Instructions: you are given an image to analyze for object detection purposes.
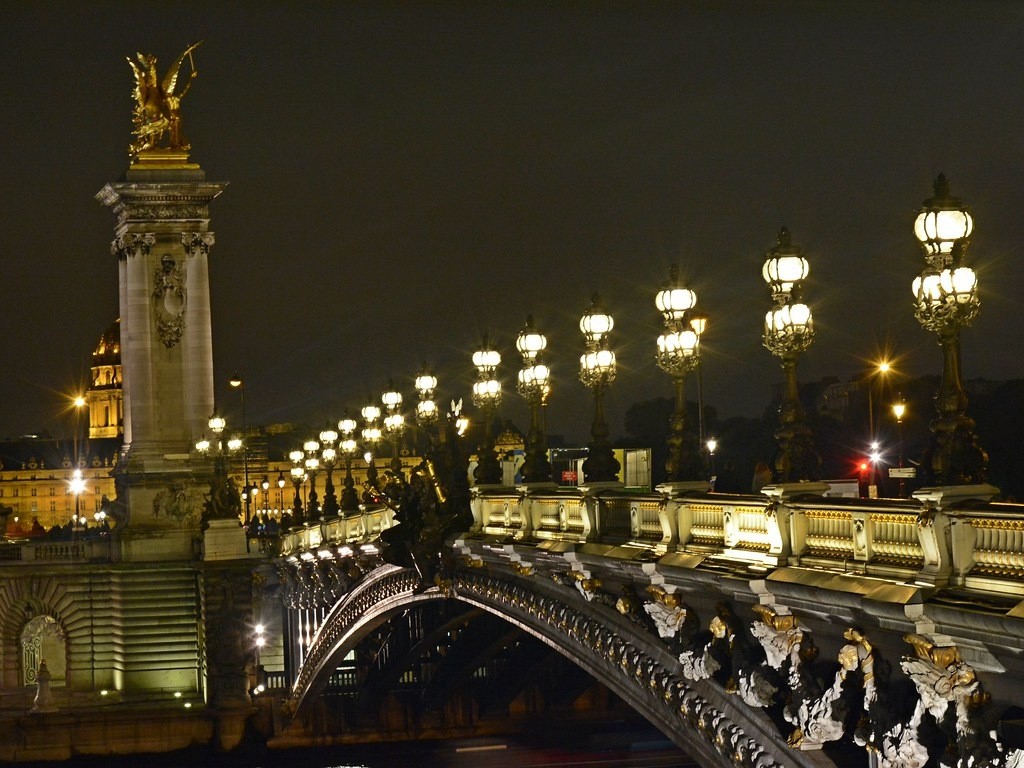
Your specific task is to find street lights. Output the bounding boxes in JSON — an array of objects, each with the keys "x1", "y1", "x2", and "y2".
[
  {"x1": 289, "y1": 449, "x2": 305, "y2": 525},
  {"x1": 228, "y1": 371, "x2": 251, "y2": 522},
  {"x1": 381, "y1": 382, "x2": 406, "y2": 487},
  {"x1": 361, "y1": 400, "x2": 383, "y2": 503},
  {"x1": 415, "y1": 363, "x2": 438, "y2": 456},
  {"x1": 277, "y1": 476, "x2": 285, "y2": 516},
  {"x1": 303, "y1": 438, "x2": 321, "y2": 521},
  {"x1": 905, "y1": 171, "x2": 999, "y2": 485},
  {"x1": 514, "y1": 313, "x2": 555, "y2": 482},
  {"x1": 577, "y1": 291, "x2": 624, "y2": 482},
  {"x1": 470, "y1": 331, "x2": 504, "y2": 483},
  {"x1": 338, "y1": 415, "x2": 360, "y2": 509},
  {"x1": 251, "y1": 484, "x2": 259, "y2": 520},
  {"x1": 654, "y1": 263, "x2": 703, "y2": 480},
  {"x1": 318, "y1": 425, "x2": 341, "y2": 520},
  {"x1": 195, "y1": 413, "x2": 242, "y2": 512},
  {"x1": 262, "y1": 477, "x2": 270, "y2": 517},
  {"x1": 759, "y1": 225, "x2": 823, "y2": 482},
  {"x1": 241, "y1": 489, "x2": 249, "y2": 525}
]
